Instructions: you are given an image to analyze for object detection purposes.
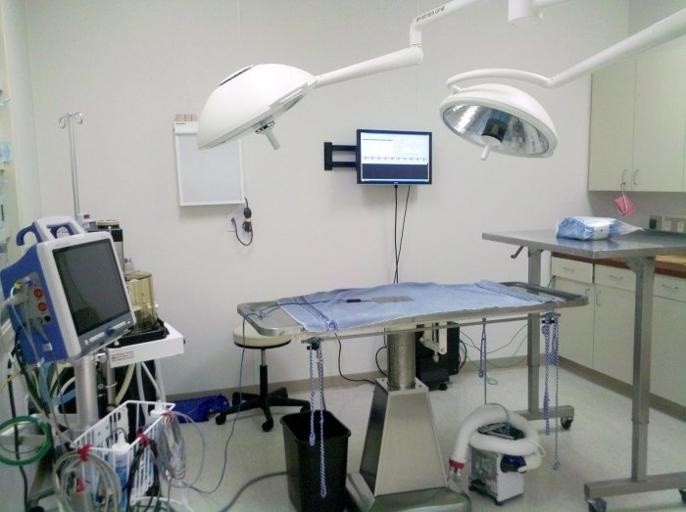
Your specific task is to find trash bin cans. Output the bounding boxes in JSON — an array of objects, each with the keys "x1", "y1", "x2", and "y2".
[{"x1": 280, "y1": 410, "x2": 351, "y2": 512}]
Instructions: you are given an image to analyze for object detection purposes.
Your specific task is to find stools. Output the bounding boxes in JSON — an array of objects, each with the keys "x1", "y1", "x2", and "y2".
[{"x1": 215, "y1": 326, "x2": 310, "y2": 432}]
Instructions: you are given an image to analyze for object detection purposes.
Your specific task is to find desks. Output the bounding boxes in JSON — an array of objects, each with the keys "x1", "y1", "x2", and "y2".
[{"x1": 482, "y1": 230, "x2": 685, "y2": 512}]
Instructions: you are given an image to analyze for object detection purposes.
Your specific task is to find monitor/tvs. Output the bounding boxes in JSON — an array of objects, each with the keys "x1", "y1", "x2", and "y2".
[
  {"x1": 0, "y1": 231, "x2": 136, "y2": 365},
  {"x1": 356, "y1": 128, "x2": 433, "y2": 185}
]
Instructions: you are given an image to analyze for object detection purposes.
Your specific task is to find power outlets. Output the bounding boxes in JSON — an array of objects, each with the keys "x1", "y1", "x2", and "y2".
[{"x1": 228, "y1": 213, "x2": 241, "y2": 232}]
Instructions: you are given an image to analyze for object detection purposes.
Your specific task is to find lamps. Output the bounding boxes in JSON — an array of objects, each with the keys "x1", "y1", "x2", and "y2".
[
  {"x1": 197, "y1": 0, "x2": 498, "y2": 152},
  {"x1": 440, "y1": 1, "x2": 686, "y2": 161}
]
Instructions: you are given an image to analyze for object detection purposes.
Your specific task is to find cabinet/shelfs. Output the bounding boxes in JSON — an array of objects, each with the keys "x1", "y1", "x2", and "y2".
[
  {"x1": 551, "y1": 255, "x2": 686, "y2": 407},
  {"x1": 587, "y1": 34, "x2": 686, "y2": 193}
]
